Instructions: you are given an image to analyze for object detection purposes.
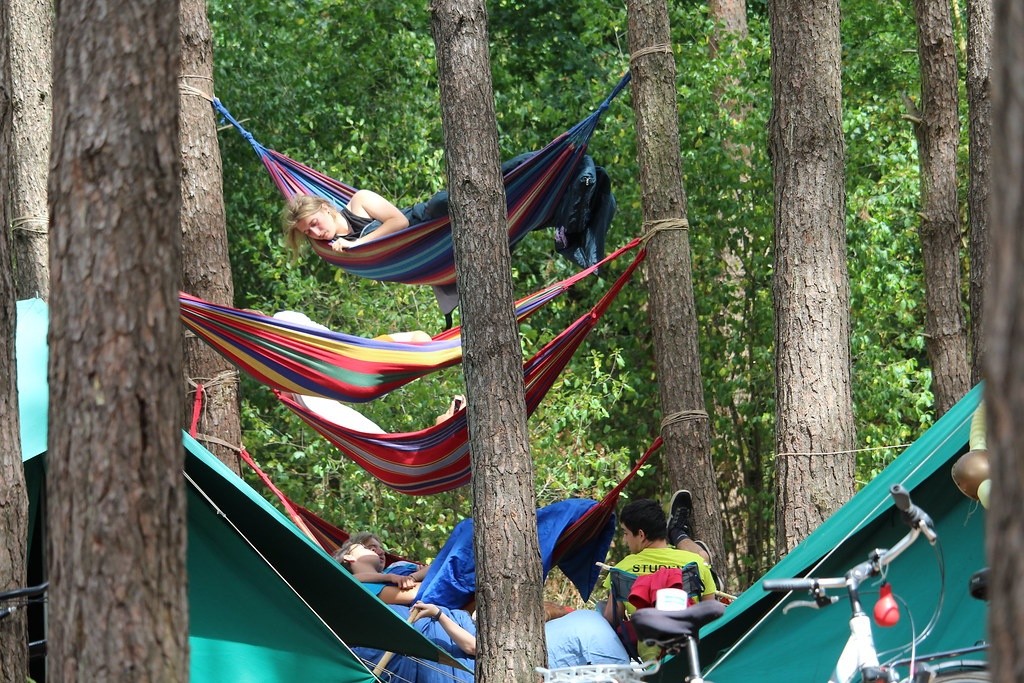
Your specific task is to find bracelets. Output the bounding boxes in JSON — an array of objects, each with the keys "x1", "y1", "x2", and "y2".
[
  {"x1": 431, "y1": 608, "x2": 443, "y2": 622},
  {"x1": 409, "y1": 574, "x2": 416, "y2": 582}
]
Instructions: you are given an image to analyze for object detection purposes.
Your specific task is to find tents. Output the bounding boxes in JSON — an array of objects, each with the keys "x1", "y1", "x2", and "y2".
[{"x1": 14, "y1": 294, "x2": 990, "y2": 683}]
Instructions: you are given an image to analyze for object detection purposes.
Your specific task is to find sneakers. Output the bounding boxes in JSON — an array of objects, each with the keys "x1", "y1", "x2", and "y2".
[
  {"x1": 666, "y1": 490, "x2": 692, "y2": 544},
  {"x1": 693, "y1": 541, "x2": 724, "y2": 592}
]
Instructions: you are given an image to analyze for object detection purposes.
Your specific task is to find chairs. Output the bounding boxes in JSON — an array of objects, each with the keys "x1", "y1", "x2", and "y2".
[{"x1": 610, "y1": 561, "x2": 704, "y2": 660}]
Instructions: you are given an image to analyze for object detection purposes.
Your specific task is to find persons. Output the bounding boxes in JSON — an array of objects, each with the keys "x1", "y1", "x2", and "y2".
[
  {"x1": 331, "y1": 530, "x2": 645, "y2": 683},
  {"x1": 604, "y1": 490, "x2": 723, "y2": 678},
  {"x1": 292, "y1": 394, "x2": 468, "y2": 433},
  {"x1": 284, "y1": 149, "x2": 542, "y2": 264},
  {"x1": 236, "y1": 309, "x2": 433, "y2": 343}
]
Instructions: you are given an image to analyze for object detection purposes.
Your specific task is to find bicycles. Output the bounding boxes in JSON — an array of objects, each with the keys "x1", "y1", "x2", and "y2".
[{"x1": 534, "y1": 484, "x2": 992, "y2": 683}]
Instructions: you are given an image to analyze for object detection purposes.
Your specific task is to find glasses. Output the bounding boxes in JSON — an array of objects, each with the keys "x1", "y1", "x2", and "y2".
[{"x1": 348, "y1": 542, "x2": 367, "y2": 555}]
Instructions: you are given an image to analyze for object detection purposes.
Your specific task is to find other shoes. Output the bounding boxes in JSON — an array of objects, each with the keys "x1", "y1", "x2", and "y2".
[{"x1": 970, "y1": 567, "x2": 991, "y2": 601}]
